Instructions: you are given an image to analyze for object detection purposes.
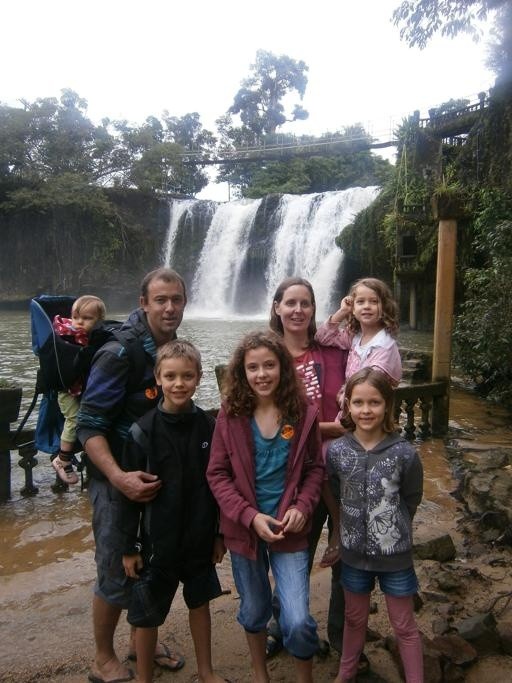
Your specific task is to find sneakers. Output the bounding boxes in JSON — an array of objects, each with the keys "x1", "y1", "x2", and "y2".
[{"x1": 52, "y1": 455, "x2": 78, "y2": 483}]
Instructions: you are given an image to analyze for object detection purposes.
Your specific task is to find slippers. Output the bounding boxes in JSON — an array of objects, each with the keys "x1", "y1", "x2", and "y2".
[
  {"x1": 266, "y1": 635, "x2": 283, "y2": 659},
  {"x1": 88, "y1": 668, "x2": 136, "y2": 683},
  {"x1": 357, "y1": 652, "x2": 369, "y2": 674},
  {"x1": 128, "y1": 643, "x2": 185, "y2": 671},
  {"x1": 316, "y1": 639, "x2": 330, "y2": 659}
]
